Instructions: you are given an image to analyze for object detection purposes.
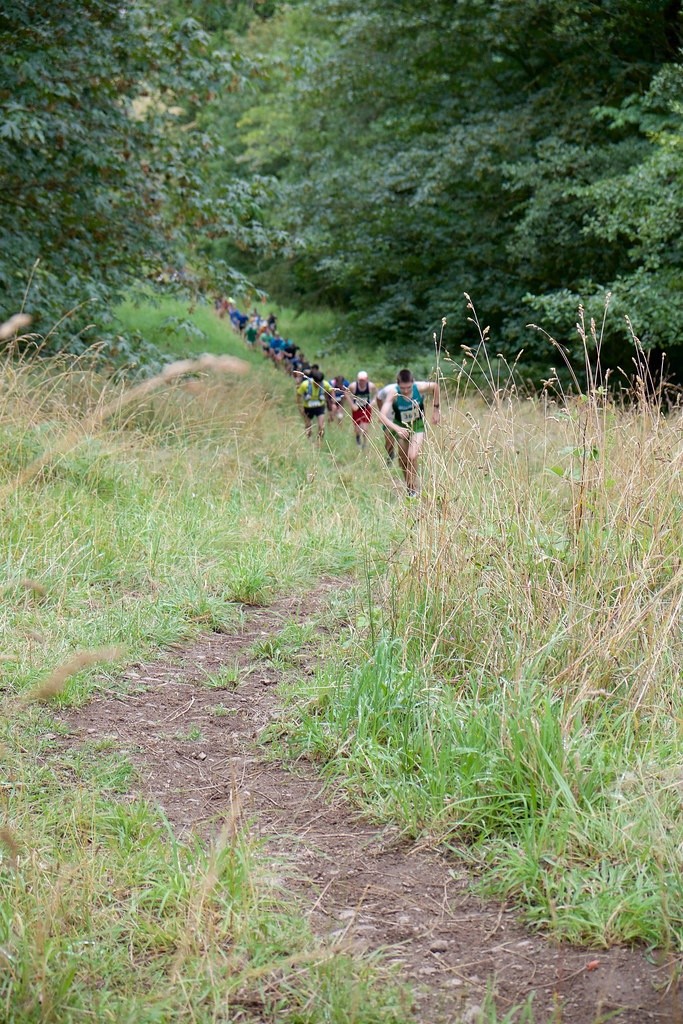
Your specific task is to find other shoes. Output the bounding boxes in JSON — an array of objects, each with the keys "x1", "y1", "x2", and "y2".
[
  {"x1": 356, "y1": 436, "x2": 361, "y2": 445},
  {"x1": 405, "y1": 490, "x2": 416, "y2": 499},
  {"x1": 385, "y1": 453, "x2": 395, "y2": 468}
]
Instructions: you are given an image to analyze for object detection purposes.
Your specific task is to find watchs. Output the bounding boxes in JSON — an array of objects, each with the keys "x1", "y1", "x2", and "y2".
[{"x1": 433, "y1": 404, "x2": 441, "y2": 409}]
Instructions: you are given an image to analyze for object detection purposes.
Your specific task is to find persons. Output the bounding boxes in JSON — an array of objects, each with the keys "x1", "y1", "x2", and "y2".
[
  {"x1": 374, "y1": 380, "x2": 408, "y2": 466},
  {"x1": 378, "y1": 368, "x2": 442, "y2": 498},
  {"x1": 214, "y1": 291, "x2": 378, "y2": 450}
]
